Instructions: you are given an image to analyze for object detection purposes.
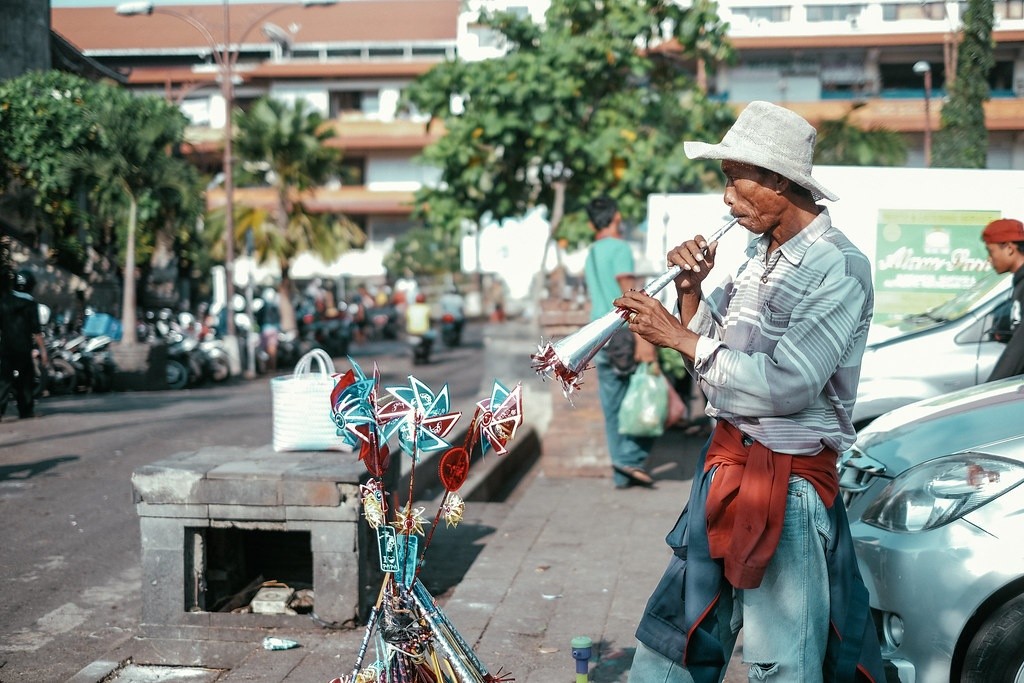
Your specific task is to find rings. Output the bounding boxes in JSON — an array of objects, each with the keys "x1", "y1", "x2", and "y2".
[{"x1": 629, "y1": 312, "x2": 638, "y2": 323}]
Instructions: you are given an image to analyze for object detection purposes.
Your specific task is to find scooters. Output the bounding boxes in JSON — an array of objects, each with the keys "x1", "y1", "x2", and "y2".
[
  {"x1": 439, "y1": 313, "x2": 466, "y2": 349},
  {"x1": 406, "y1": 316, "x2": 439, "y2": 367},
  {"x1": 1, "y1": 294, "x2": 397, "y2": 403}
]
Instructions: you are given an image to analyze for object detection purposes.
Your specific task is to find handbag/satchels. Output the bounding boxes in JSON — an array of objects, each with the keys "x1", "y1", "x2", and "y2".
[
  {"x1": 616, "y1": 361, "x2": 686, "y2": 437},
  {"x1": 608, "y1": 331, "x2": 636, "y2": 373}
]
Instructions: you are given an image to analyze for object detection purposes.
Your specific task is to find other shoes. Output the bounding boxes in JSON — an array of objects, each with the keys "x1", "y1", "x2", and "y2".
[{"x1": 614, "y1": 463, "x2": 653, "y2": 485}]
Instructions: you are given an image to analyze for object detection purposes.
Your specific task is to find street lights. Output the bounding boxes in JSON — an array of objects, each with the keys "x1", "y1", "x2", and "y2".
[{"x1": 115, "y1": 1, "x2": 339, "y2": 338}]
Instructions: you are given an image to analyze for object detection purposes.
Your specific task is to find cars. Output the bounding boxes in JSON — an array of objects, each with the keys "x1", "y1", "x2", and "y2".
[
  {"x1": 850, "y1": 268, "x2": 1024, "y2": 434},
  {"x1": 833, "y1": 375, "x2": 1024, "y2": 683}
]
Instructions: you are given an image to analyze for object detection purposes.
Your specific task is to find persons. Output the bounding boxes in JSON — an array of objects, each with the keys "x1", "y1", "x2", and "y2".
[
  {"x1": 611, "y1": 101, "x2": 873, "y2": 683},
  {"x1": 584, "y1": 196, "x2": 656, "y2": 489},
  {"x1": 981, "y1": 219, "x2": 1024, "y2": 343},
  {"x1": 264, "y1": 267, "x2": 466, "y2": 346},
  {"x1": 0, "y1": 270, "x2": 47, "y2": 417}
]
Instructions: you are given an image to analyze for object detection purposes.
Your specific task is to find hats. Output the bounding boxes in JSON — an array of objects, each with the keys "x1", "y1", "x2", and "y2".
[
  {"x1": 684, "y1": 99, "x2": 839, "y2": 203},
  {"x1": 982, "y1": 218, "x2": 1024, "y2": 243}
]
